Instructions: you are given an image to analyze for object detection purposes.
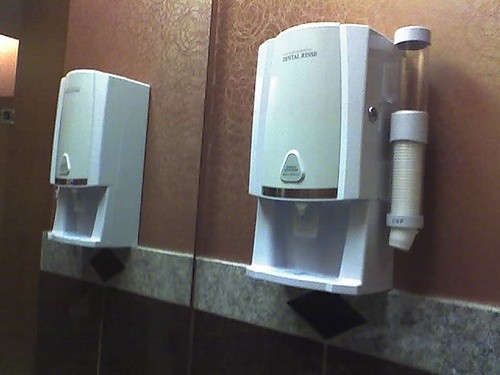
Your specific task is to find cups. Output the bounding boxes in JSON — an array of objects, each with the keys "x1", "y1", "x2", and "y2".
[{"x1": 388, "y1": 141, "x2": 424, "y2": 252}]
